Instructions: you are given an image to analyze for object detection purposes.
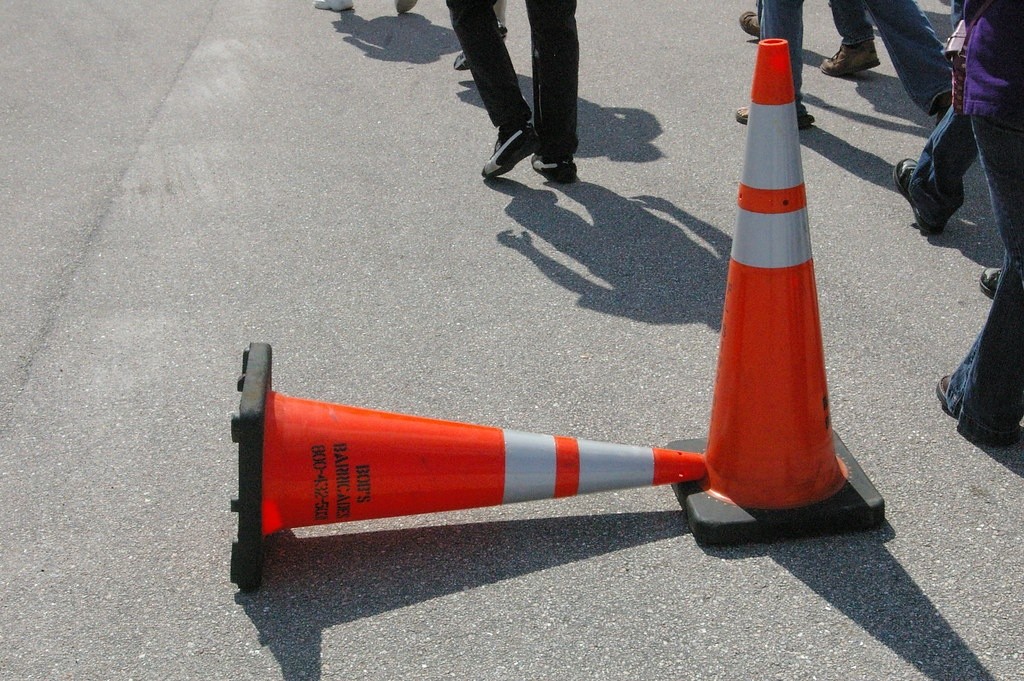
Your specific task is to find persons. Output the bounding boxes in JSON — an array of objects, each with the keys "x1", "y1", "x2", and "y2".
[
  {"x1": 446, "y1": 0, "x2": 579, "y2": 183},
  {"x1": 736, "y1": 0, "x2": 1024, "y2": 447},
  {"x1": 314, "y1": 0, "x2": 508, "y2": 74}
]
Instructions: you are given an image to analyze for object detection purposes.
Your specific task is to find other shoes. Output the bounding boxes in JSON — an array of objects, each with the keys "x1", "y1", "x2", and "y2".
[
  {"x1": 936, "y1": 374, "x2": 1022, "y2": 448},
  {"x1": 820, "y1": 38, "x2": 881, "y2": 76},
  {"x1": 935, "y1": 90, "x2": 952, "y2": 126},
  {"x1": 739, "y1": 11, "x2": 760, "y2": 37}
]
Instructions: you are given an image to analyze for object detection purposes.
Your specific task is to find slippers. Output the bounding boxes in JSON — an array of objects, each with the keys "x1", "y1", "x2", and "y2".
[{"x1": 735, "y1": 101, "x2": 815, "y2": 128}]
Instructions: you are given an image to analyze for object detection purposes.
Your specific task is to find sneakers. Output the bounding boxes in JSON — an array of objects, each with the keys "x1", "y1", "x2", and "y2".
[
  {"x1": 531, "y1": 154, "x2": 578, "y2": 184},
  {"x1": 893, "y1": 158, "x2": 947, "y2": 236},
  {"x1": 979, "y1": 266, "x2": 1002, "y2": 300},
  {"x1": 481, "y1": 123, "x2": 544, "y2": 179},
  {"x1": 313, "y1": 0, "x2": 354, "y2": 12},
  {"x1": 453, "y1": 16, "x2": 508, "y2": 71},
  {"x1": 394, "y1": 0, "x2": 419, "y2": 14}
]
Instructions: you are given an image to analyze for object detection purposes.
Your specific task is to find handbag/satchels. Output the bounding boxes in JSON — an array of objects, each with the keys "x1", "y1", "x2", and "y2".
[{"x1": 943, "y1": 19, "x2": 967, "y2": 116}]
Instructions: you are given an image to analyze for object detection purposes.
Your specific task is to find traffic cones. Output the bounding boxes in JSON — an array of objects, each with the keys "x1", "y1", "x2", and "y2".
[
  {"x1": 228, "y1": 343, "x2": 714, "y2": 587},
  {"x1": 655, "y1": 37, "x2": 886, "y2": 544}
]
